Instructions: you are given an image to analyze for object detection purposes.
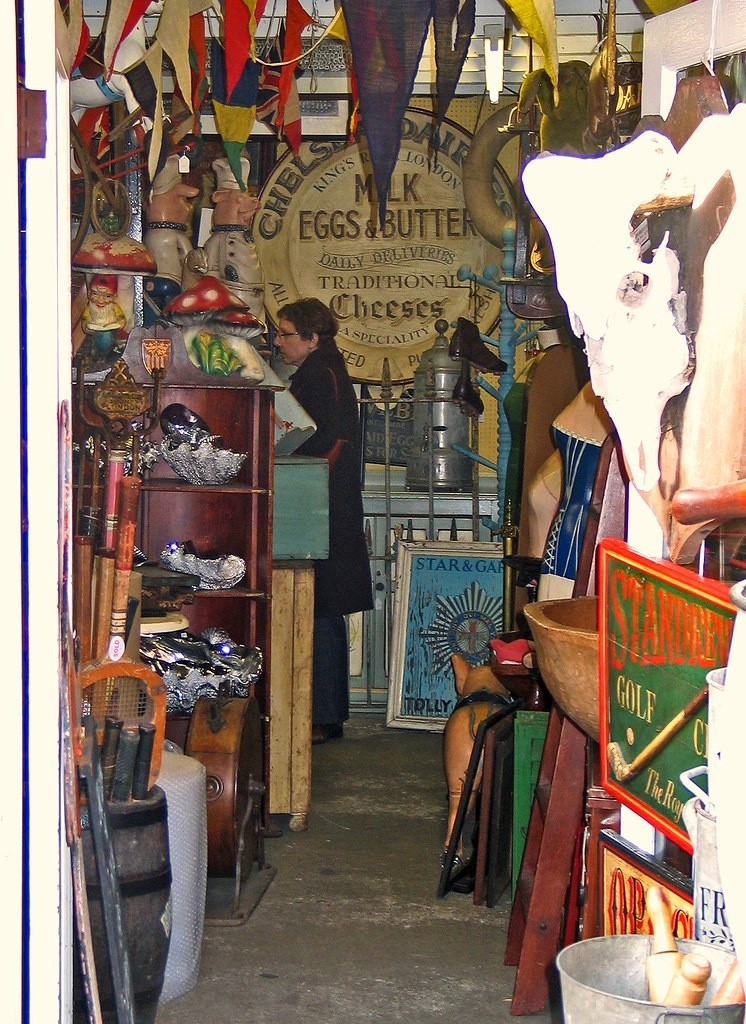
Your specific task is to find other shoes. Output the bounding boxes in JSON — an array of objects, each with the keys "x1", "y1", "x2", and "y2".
[{"x1": 312, "y1": 722, "x2": 344, "y2": 744}]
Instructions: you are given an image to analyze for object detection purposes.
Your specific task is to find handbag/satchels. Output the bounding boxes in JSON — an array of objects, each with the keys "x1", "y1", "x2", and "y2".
[{"x1": 294, "y1": 368, "x2": 350, "y2": 472}]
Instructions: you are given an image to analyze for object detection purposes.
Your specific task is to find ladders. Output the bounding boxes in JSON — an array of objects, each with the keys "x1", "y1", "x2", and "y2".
[{"x1": 502, "y1": 417, "x2": 637, "y2": 1016}]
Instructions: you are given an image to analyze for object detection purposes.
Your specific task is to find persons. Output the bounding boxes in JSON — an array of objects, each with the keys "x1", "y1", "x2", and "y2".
[
  {"x1": 81, "y1": 276, "x2": 127, "y2": 357},
  {"x1": 274, "y1": 297, "x2": 376, "y2": 743}
]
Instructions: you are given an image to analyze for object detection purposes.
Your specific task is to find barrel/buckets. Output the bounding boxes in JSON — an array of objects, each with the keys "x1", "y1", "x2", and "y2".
[{"x1": 557, "y1": 932, "x2": 746, "y2": 1024}]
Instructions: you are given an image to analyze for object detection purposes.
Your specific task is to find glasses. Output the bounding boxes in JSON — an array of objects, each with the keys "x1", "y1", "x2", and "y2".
[{"x1": 276, "y1": 329, "x2": 321, "y2": 340}]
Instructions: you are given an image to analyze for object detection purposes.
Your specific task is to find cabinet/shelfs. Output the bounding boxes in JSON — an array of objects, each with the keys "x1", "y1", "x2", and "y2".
[
  {"x1": 74, "y1": 324, "x2": 286, "y2": 929},
  {"x1": 344, "y1": 492, "x2": 500, "y2": 715}
]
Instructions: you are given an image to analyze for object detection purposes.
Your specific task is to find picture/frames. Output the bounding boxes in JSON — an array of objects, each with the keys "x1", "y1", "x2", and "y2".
[
  {"x1": 473, "y1": 713, "x2": 514, "y2": 908},
  {"x1": 386, "y1": 539, "x2": 505, "y2": 733}
]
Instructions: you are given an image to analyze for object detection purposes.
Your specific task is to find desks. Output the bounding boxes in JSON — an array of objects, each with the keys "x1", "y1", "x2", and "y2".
[{"x1": 490, "y1": 632, "x2": 553, "y2": 711}]
